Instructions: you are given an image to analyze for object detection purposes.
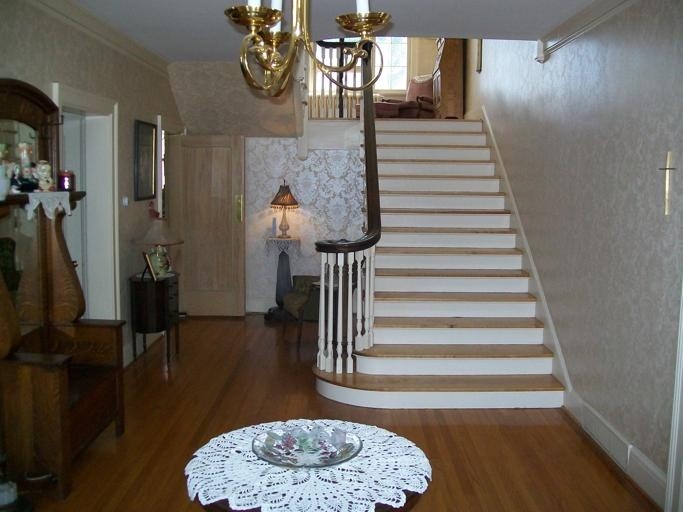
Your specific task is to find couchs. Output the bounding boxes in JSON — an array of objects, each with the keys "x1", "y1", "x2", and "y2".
[
  {"x1": 382, "y1": 74, "x2": 433, "y2": 119},
  {"x1": 356, "y1": 103, "x2": 400, "y2": 118}
]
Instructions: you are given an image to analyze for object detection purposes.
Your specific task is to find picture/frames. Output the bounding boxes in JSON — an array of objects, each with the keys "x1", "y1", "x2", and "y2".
[
  {"x1": 138, "y1": 251, "x2": 158, "y2": 283},
  {"x1": 133, "y1": 119, "x2": 157, "y2": 202}
]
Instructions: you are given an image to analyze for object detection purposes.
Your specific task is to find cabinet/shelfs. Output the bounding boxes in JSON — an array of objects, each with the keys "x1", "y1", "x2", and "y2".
[
  {"x1": 1, "y1": 191, "x2": 86, "y2": 218},
  {"x1": 432, "y1": 38, "x2": 464, "y2": 119},
  {"x1": 129, "y1": 273, "x2": 180, "y2": 363}
]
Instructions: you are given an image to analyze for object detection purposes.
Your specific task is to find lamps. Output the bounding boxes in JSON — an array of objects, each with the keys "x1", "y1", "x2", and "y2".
[
  {"x1": 269, "y1": 180, "x2": 300, "y2": 239},
  {"x1": 223, "y1": 0, "x2": 389, "y2": 105},
  {"x1": 130, "y1": 219, "x2": 186, "y2": 275}
]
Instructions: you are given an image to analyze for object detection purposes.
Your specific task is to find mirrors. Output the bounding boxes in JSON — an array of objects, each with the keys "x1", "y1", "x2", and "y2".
[{"x1": 0, "y1": 119, "x2": 46, "y2": 337}]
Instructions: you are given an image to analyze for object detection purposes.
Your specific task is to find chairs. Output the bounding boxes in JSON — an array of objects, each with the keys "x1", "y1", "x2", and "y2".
[{"x1": 282, "y1": 239, "x2": 366, "y2": 354}]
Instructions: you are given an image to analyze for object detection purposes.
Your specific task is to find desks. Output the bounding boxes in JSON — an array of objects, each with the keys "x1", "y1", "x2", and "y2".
[{"x1": 6, "y1": 319, "x2": 126, "y2": 501}]
